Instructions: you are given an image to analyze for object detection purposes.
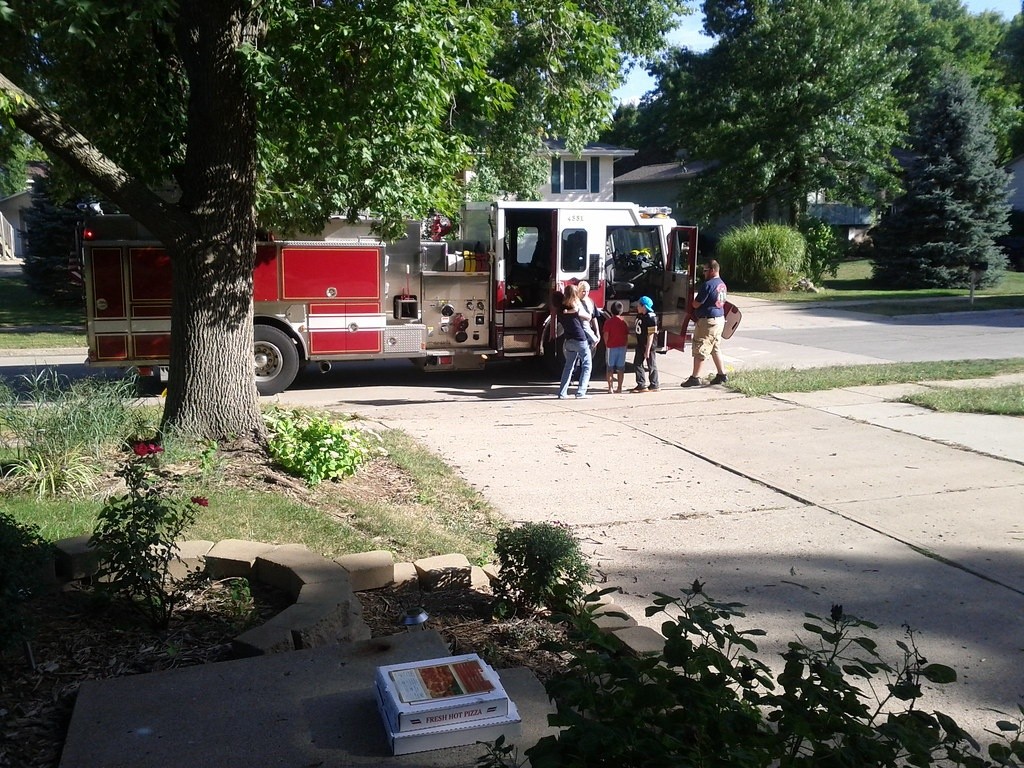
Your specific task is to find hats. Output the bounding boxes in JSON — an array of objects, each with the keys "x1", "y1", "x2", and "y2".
[{"x1": 640, "y1": 296, "x2": 653, "y2": 312}]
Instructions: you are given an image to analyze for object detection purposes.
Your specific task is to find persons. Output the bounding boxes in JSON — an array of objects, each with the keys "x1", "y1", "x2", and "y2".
[
  {"x1": 602, "y1": 301, "x2": 630, "y2": 394},
  {"x1": 680, "y1": 259, "x2": 729, "y2": 387},
  {"x1": 551, "y1": 280, "x2": 601, "y2": 399},
  {"x1": 630, "y1": 296, "x2": 661, "y2": 393}
]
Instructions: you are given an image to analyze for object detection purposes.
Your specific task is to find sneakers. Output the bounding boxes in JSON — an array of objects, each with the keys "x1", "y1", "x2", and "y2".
[
  {"x1": 681, "y1": 376, "x2": 700, "y2": 387},
  {"x1": 710, "y1": 373, "x2": 727, "y2": 385}
]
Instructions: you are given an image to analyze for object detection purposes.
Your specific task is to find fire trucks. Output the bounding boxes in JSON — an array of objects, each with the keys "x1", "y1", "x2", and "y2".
[{"x1": 78, "y1": 200, "x2": 699, "y2": 396}]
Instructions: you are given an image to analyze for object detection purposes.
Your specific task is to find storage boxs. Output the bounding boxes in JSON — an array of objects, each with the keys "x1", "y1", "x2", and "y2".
[
  {"x1": 373, "y1": 681, "x2": 522, "y2": 755},
  {"x1": 375, "y1": 651, "x2": 508, "y2": 732}
]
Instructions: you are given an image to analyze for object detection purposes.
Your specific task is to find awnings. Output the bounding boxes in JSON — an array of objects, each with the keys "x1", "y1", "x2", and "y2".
[{"x1": 807, "y1": 203, "x2": 873, "y2": 227}]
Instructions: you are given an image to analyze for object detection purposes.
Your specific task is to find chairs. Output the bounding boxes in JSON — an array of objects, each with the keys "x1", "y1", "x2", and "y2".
[{"x1": 606, "y1": 253, "x2": 634, "y2": 291}]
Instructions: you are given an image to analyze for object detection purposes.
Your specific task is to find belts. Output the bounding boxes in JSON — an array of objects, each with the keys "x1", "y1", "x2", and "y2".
[{"x1": 699, "y1": 316, "x2": 714, "y2": 318}]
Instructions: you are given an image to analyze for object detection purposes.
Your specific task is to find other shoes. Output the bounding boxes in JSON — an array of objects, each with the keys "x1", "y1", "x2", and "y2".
[
  {"x1": 559, "y1": 394, "x2": 575, "y2": 399},
  {"x1": 630, "y1": 388, "x2": 649, "y2": 392},
  {"x1": 575, "y1": 393, "x2": 592, "y2": 398},
  {"x1": 648, "y1": 388, "x2": 660, "y2": 391}
]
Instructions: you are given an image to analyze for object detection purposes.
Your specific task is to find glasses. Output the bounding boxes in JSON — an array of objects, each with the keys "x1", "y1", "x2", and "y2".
[{"x1": 704, "y1": 268, "x2": 716, "y2": 273}]
